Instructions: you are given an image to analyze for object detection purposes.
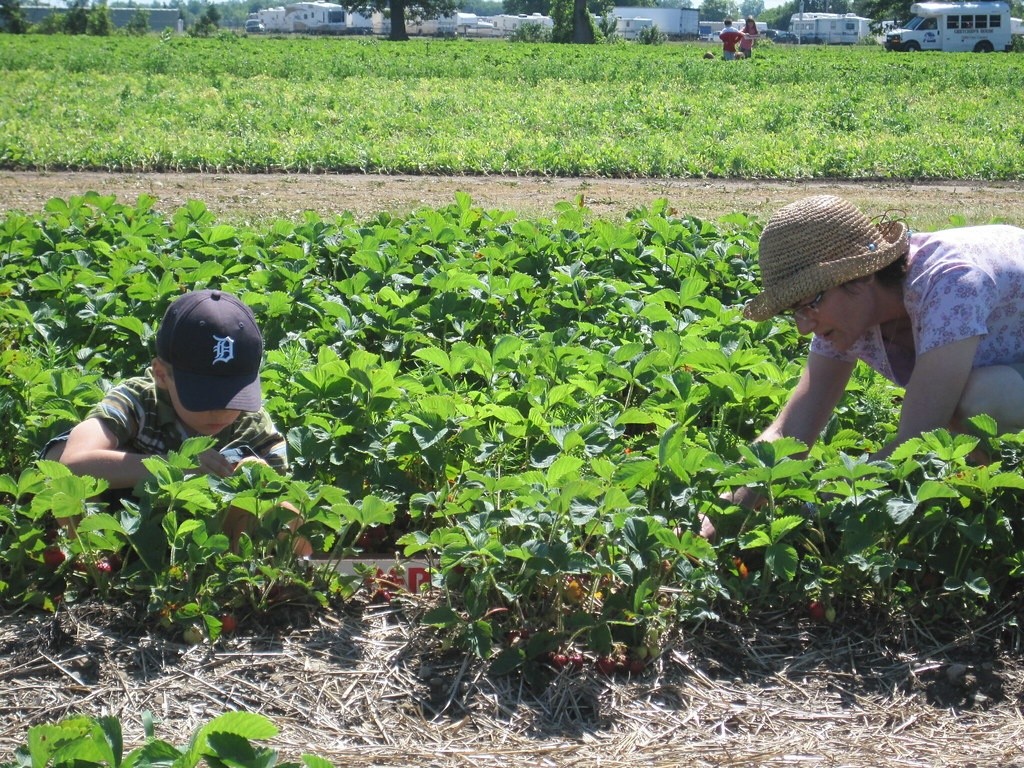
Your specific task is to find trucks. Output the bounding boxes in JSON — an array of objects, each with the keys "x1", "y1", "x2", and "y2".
[{"x1": 789, "y1": 16, "x2": 875, "y2": 45}]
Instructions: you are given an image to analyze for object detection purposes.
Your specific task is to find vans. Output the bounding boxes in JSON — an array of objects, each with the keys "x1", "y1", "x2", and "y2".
[{"x1": 884, "y1": 1, "x2": 1013, "y2": 53}]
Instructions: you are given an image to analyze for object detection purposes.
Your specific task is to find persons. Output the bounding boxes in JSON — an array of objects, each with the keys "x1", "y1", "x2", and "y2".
[
  {"x1": 719, "y1": 20, "x2": 743, "y2": 61},
  {"x1": 44, "y1": 288, "x2": 289, "y2": 499},
  {"x1": 738, "y1": 19, "x2": 758, "y2": 59},
  {"x1": 701, "y1": 194, "x2": 1023, "y2": 464}
]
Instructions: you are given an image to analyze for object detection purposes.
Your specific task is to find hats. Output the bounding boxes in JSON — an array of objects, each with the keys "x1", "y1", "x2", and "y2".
[
  {"x1": 157, "y1": 290, "x2": 262, "y2": 412},
  {"x1": 743, "y1": 195, "x2": 912, "y2": 322}
]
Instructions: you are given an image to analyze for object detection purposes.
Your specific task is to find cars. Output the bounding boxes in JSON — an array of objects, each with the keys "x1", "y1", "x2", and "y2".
[
  {"x1": 773, "y1": 32, "x2": 799, "y2": 45},
  {"x1": 707, "y1": 30, "x2": 724, "y2": 44},
  {"x1": 800, "y1": 32, "x2": 827, "y2": 45},
  {"x1": 760, "y1": 29, "x2": 779, "y2": 40},
  {"x1": 245, "y1": 18, "x2": 264, "y2": 33}
]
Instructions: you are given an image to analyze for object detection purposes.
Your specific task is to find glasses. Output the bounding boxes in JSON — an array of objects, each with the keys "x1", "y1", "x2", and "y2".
[{"x1": 777, "y1": 290, "x2": 824, "y2": 324}]
